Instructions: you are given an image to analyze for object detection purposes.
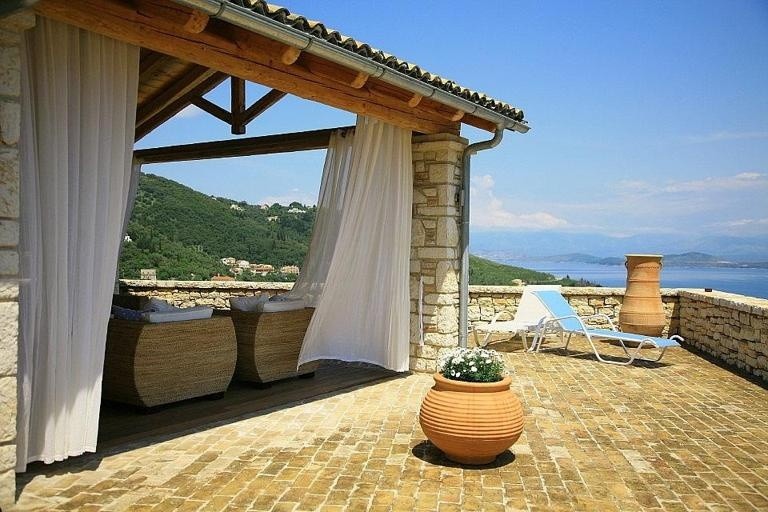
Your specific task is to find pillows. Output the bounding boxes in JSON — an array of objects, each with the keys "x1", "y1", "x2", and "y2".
[
  {"x1": 112, "y1": 296, "x2": 213, "y2": 322},
  {"x1": 229, "y1": 291, "x2": 306, "y2": 312}
]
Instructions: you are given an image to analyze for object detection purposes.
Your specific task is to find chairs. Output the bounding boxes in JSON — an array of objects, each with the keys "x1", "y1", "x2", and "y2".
[
  {"x1": 531, "y1": 290, "x2": 685, "y2": 365},
  {"x1": 215, "y1": 307, "x2": 319, "y2": 390},
  {"x1": 472, "y1": 284, "x2": 562, "y2": 351},
  {"x1": 103, "y1": 313, "x2": 238, "y2": 414}
]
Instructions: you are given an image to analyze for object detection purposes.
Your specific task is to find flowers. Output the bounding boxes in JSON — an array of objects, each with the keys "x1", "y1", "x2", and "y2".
[{"x1": 436, "y1": 346, "x2": 510, "y2": 382}]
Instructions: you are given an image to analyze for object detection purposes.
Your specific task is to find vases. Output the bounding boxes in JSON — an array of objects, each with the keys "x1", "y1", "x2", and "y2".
[
  {"x1": 419, "y1": 371, "x2": 525, "y2": 465},
  {"x1": 618, "y1": 253, "x2": 667, "y2": 347}
]
